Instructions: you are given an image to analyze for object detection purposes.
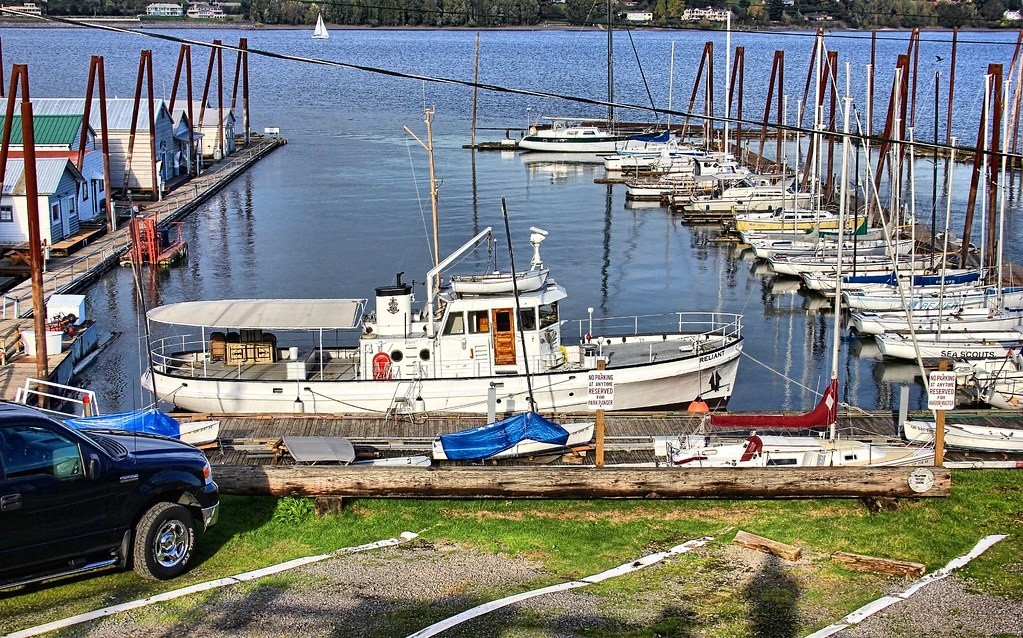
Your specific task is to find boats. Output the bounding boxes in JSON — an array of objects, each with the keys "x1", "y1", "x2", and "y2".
[
  {"x1": 906, "y1": 420, "x2": 1023, "y2": 455},
  {"x1": 122, "y1": 210, "x2": 189, "y2": 267}
]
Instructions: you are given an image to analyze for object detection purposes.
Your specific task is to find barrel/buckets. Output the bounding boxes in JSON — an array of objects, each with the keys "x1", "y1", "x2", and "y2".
[{"x1": 289, "y1": 346, "x2": 298, "y2": 360}]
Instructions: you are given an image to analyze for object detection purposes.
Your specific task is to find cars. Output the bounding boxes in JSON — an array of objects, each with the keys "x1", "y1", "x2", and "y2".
[{"x1": 0, "y1": 399, "x2": 220, "y2": 592}]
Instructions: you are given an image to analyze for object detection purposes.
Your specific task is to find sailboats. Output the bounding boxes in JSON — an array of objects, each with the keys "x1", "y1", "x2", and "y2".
[
  {"x1": 141, "y1": 82, "x2": 745, "y2": 408},
  {"x1": 517, "y1": 0, "x2": 1023, "y2": 410},
  {"x1": 311, "y1": 13, "x2": 330, "y2": 39},
  {"x1": 431, "y1": 196, "x2": 596, "y2": 464},
  {"x1": 656, "y1": 63, "x2": 946, "y2": 467}
]
